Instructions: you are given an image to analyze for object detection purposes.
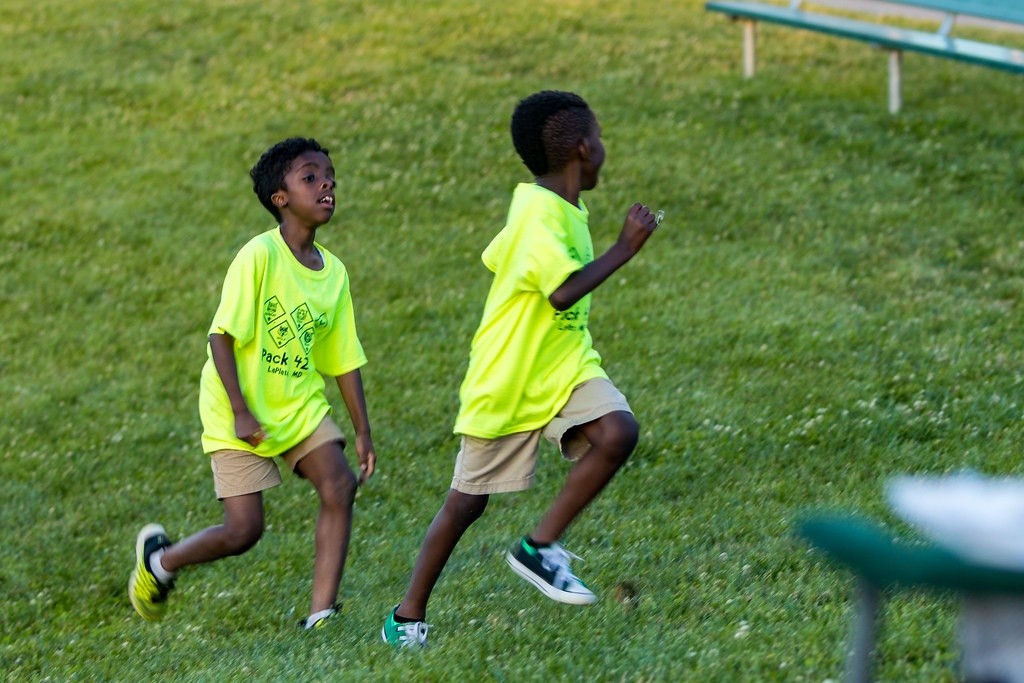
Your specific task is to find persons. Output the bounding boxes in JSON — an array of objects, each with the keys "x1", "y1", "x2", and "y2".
[
  {"x1": 381, "y1": 90, "x2": 658, "y2": 643},
  {"x1": 128, "y1": 135, "x2": 375, "y2": 631}
]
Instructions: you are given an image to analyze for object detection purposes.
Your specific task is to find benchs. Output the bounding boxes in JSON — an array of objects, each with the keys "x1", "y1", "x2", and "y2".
[{"x1": 704, "y1": 0, "x2": 1024, "y2": 113}]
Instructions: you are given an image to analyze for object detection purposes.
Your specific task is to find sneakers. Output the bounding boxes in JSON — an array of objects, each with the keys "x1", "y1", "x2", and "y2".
[
  {"x1": 128, "y1": 523, "x2": 178, "y2": 621},
  {"x1": 504, "y1": 531, "x2": 598, "y2": 605},
  {"x1": 298, "y1": 602, "x2": 343, "y2": 629},
  {"x1": 381, "y1": 604, "x2": 434, "y2": 648}
]
